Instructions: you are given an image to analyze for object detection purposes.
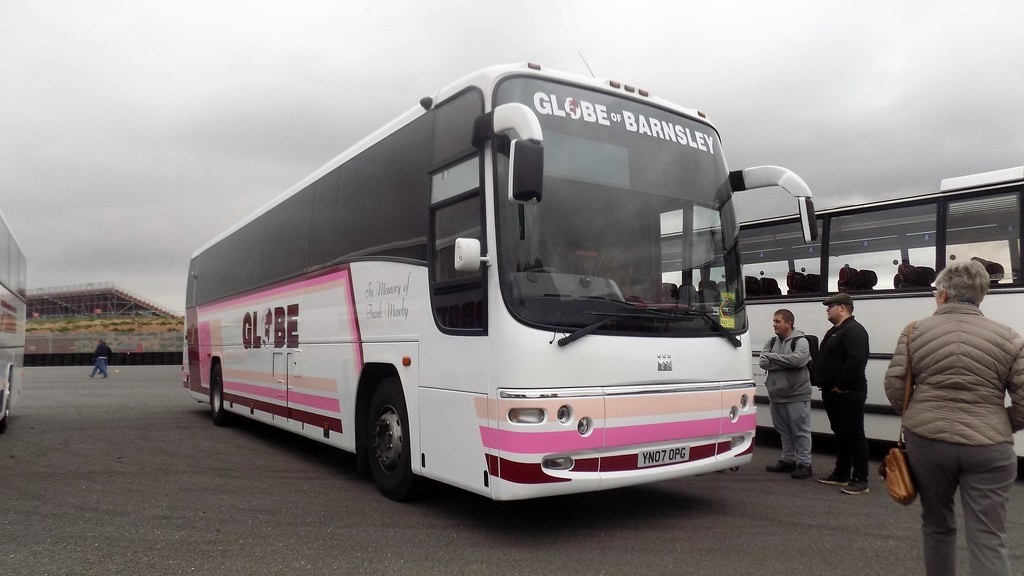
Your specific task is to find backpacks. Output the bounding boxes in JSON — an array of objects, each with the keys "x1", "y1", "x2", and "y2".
[{"x1": 769, "y1": 335, "x2": 818, "y2": 386}]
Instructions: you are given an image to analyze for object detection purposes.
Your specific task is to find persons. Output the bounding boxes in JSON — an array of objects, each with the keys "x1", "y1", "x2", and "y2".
[
  {"x1": 89, "y1": 339, "x2": 113, "y2": 379},
  {"x1": 810, "y1": 291, "x2": 869, "y2": 495},
  {"x1": 760, "y1": 309, "x2": 815, "y2": 479},
  {"x1": 884, "y1": 259, "x2": 1024, "y2": 576}
]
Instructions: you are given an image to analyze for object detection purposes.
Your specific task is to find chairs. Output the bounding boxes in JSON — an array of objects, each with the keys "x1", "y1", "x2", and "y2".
[{"x1": 661, "y1": 256, "x2": 1005, "y2": 305}]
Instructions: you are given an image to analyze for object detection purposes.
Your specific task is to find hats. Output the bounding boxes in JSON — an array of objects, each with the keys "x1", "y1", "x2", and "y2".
[{"x1": 822, "y1": 293, "x2": 853, "y2": 306}]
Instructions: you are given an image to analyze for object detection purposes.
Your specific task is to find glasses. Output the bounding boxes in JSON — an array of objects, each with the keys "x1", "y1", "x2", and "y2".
[
  {"x1": 932, "y1": 290, "x2": 941, "y2": 296},
  {"x1": 828, "y1": 304, "x2": 840, "y2": 308}
]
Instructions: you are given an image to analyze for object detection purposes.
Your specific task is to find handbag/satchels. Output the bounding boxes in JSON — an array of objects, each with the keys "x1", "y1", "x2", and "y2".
[{"x1": 877, "y1": 448, "x2": 917, "y2": 507}]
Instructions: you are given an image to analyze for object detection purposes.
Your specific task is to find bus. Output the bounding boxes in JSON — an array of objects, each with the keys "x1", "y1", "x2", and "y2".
[
  {"x1": 182, "y1": 61, "x2": 817, "y2": 503},
  {"x1": 659, "y1": 166, "x2": 1024, "y2": 458},
  {"x1": 0, "y1": 211, "x2": 27, "y2": 431}
]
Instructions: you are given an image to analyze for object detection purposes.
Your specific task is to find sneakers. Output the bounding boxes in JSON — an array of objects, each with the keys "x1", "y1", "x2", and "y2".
[
  {"x1": 839, "y1": 479, "x2": 869, "y2": 494},
  {"x1": 791, "y1": 464, "x2": 813, "y2": 479},
  {"x1": 766, "y1": 460, "x2": 797, "y2": 473},
  {"x1": 818, "y1": 471, "x2": 848, "y2": 486}
]
